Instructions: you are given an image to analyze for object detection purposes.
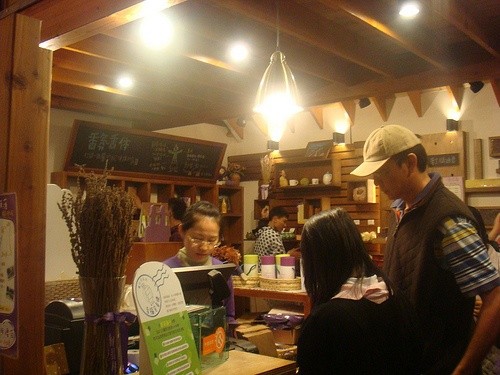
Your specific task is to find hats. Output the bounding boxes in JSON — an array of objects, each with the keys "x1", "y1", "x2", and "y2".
[{"x1": 350, "y1": 124, "x2": 421, "y2": 177}]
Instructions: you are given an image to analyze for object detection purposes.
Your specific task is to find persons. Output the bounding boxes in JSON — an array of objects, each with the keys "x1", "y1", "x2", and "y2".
[
  {"x1": 349, "y1": 124, "x2": 500, "y2": 375},
  {"x1": 255, "y1": 207, "x2": 301, "y2": 273},
  {"x1": 163, "y1": 197, "x2": 235, "y2": 320},
  {"x1": 467, "y1": 205, "x2": 500, "y2": 375},
  {"x1": 296, "y1": 207, "x2": 433, "y2": 375}
]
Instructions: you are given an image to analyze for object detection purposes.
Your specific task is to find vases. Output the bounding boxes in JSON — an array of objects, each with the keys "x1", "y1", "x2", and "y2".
[
  {"x1": 216, "y1": 180, "x2": 225, "y2": 185},
  {"x1": 230, "y1": 173, "x2": 241, "y2": 187},
  {"x1": 76, "y1": 276, "x2": 126, "y2": 375}
]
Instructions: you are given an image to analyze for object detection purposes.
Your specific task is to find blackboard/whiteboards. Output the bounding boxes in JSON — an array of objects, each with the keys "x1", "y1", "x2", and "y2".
[{"x1": 63, "y1": 118, "x2": 228, "y2": 183}]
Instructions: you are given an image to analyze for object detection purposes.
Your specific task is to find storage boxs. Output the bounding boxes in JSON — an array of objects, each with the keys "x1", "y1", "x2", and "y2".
[
  {"x1": 346, "y1": 179, "x2": 376, "y2": 204},
  {"x1": 304, "y1": 196, "x2": 330, "y2": 219},
  {"x1": 243, "y1": 329, "x2": 278, "y2": 358},
  {"x1": 274, "y1": 159, "x2": 342, "y2": 191},
  {"x1": 236, "y1": 324, "x2": 270, "y2": 339},
  {"x1": 188, "y1": 303, "x2": 230, "y2": 370},
  {"x1": 272, "y1": 328, "x2": 300, "y2": 345}
]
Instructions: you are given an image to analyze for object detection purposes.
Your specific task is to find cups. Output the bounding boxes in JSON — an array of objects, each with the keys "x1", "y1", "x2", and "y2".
[
  {"x1": 323, "y1": 171, "x2": 333, "y2": 184},
  {"x1": 290, "y1": 179, "x2": 299, "y2": 185},
  {"x1": 312, "y1": 179, "x2": 320, "y2": 184}
]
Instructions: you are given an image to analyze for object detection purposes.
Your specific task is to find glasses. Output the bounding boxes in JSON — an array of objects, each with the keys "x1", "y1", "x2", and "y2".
[{"x1": 185, "y1": 231, "x2": 218, "y2": 247}]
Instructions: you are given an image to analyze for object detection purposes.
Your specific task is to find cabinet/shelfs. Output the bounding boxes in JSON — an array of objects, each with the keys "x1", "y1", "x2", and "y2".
[{"x1": 50, "y1": 170, "x2": 245, "y2": 272}]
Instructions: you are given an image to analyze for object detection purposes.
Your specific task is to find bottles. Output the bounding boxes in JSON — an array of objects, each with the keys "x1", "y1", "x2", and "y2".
[
  {"x1": 221, "y1": 198, "x2": 227, "y2": 214},
  {"x1": 243, "y1": 254, "x2": 296, "y2": 279}
]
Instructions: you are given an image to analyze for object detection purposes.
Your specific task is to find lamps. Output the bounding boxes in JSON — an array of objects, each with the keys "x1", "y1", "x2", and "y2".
[
  {"x1": 252, "y1": 0, "x2": 304, "y2": 116},
  {"x1": 266, "y1": 140, "x2": 280, "y2": 151},
  {"x1": 332, "y1": 133, "x2": 345, "y2": 144},
  {"x1": 446, "y1": 119, "x2": 459, "y2": 132}
]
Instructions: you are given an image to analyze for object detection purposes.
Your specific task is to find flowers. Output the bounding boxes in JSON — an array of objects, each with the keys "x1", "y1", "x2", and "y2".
[
  {"x1": 227, "y1": 162, "x2": 246, "y2": 177},
  {"x1": 216, "y1": 165, "x2": 229, "y2": 181}
]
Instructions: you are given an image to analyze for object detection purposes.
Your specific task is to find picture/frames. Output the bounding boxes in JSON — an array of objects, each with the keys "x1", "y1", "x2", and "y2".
[
  {"x1": 488, "y1": 136, "x2": 500, "y2": 157},
  {"x1": 304, "y1": 140, "x2": 334, "y2": 159}
]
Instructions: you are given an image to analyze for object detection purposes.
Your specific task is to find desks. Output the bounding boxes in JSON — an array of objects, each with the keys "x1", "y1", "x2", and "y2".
[
  {"x1": 233, "y1": 286, "x2": 312, "y2": 321},
  {"x1": 127, "y1": 347, "x2": 298, "y2": 375}
]
